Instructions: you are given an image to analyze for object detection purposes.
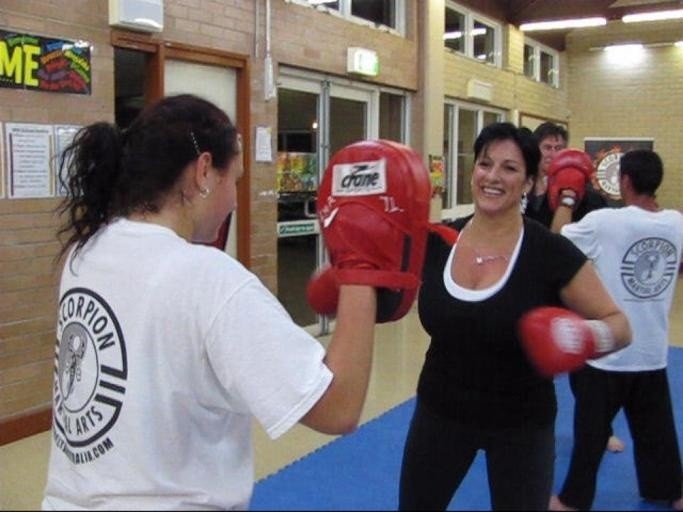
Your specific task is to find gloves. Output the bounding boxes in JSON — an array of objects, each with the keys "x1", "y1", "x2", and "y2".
[{"x1": 521, "y1": 306, "x2": 616, "y2": 378}]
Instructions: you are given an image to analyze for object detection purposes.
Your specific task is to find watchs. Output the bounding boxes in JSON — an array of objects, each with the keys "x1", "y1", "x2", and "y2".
[{"x1": 557, "y1": 194, "x2": 577, "y2": 211}]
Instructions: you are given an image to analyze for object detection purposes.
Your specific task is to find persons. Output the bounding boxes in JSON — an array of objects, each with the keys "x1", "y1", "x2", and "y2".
[
  {"x1": 548, "y1": 147, "x2": 682, "y2": 512},
  {"x1": 395, "y1": 120, "x2": 635, "y2": 511},
  {"x1": 520, "y1": 120, "x2": 624, "y2": 455},
  {"x1": 39, "y1": 93, "x2": 432, "y2": 511}
]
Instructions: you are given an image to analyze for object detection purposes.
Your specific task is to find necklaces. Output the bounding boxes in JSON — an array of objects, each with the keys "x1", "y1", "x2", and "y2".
[{"x1": 466, "y1": 214, "x2": 525, "y2": 266}]
